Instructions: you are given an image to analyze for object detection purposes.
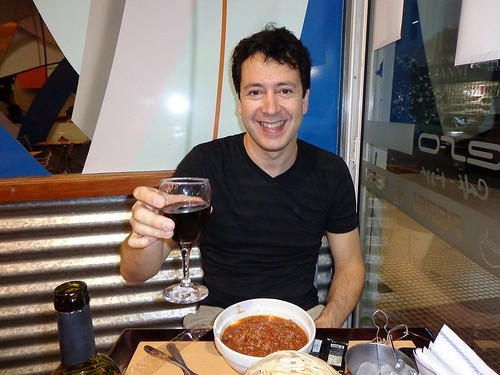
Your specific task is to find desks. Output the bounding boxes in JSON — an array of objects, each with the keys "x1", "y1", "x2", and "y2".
[{"x1": 106, "y1": 328, "x2": 435, "y2": 375}]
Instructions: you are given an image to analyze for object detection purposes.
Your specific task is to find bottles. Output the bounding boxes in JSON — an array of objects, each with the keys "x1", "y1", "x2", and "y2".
[{"x1": 51, "y1": 281, "x2": 122, "y2": 375}]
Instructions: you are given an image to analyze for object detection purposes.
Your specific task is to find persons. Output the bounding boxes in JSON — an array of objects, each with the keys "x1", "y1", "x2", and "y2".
[{"x1": 120, "y1": 28, "x2": 364, "y2": 330}]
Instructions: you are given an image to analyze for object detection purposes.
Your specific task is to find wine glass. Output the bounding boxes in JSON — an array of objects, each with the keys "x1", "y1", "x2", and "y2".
[{"x1": 156, "y1": 177, "x2": 212, "y2": 304}]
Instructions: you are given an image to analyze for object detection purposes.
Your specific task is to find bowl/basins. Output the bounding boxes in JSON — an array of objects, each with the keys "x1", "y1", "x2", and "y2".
[
  {"x1": 345, "y1": 343, "x2": 418, "y2": 375},
  {"x1": 213, "y1": 297, "x2": 316, "y2": 374}
]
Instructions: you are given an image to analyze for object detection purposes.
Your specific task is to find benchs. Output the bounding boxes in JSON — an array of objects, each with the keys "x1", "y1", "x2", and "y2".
[{"x1": 0, "y1": 169, "x2": 352, "y2": 375}]
